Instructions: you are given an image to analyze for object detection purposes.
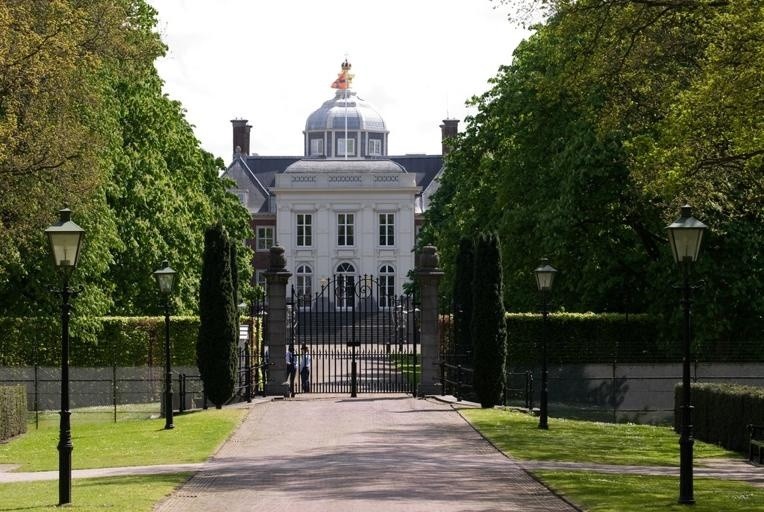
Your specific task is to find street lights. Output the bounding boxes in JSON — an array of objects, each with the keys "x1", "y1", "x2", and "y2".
[
  {"x1": 44, "y1": 208, "x2": 86, "y2": 504},
  {"x1": 532, "y1": 257, "x2": 558, "y2": 430},
  {"x1": 664, "y1": 204, "x2": 709, "y2": 506},
  {"x1": 153, "y1": 259, "x2": 177, "y2": 429}
]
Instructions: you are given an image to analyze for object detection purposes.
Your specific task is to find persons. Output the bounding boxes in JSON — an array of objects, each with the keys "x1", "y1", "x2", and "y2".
[
  {"x1": 286, "y1": 343, "x2": 298, "y2": 389},
  {"x1": 298, "y1": 341, "x2": 310, "y2": 393}
]
